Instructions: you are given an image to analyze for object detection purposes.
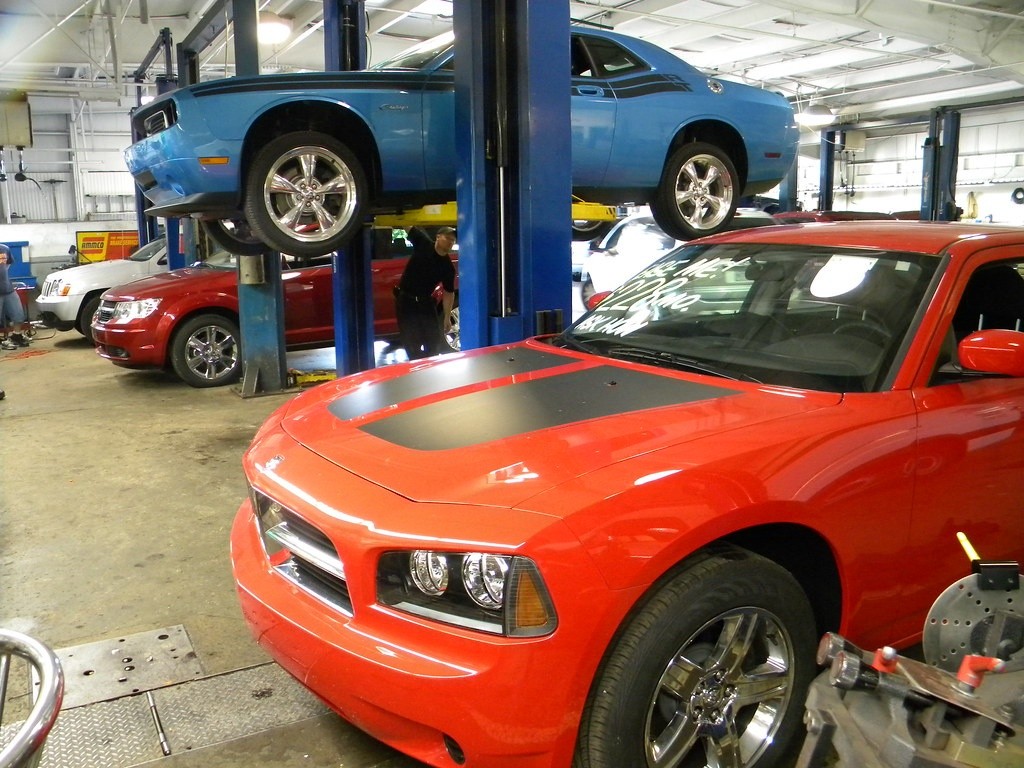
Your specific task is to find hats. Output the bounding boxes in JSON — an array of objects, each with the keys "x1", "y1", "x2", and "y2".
[{"x1": 437, "y1": 225, "x2": 457, "y2": 237}]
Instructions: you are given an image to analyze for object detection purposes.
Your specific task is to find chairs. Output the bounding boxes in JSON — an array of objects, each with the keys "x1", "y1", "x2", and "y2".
[{"x1": 952, "y1": 264, "x2": 1024, "y2": 341}]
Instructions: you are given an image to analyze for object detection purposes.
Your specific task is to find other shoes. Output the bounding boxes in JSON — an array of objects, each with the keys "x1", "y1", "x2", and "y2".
[
  {"x1": 1, "y1": 339, "x2": 17, "y2": 350},
  {"x1": 11, "y1": 331, "x2": 33, "y2": 342}
]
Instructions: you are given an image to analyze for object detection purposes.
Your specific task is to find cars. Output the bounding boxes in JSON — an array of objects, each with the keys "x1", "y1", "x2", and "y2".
[
  {"x1": 229, "y1": 214, "x2": 1023, "y2": 768},
  {"x1": 35, "y1": 223, "x2": 194, "y2": 347},
  {"x1": 91, "y1": 219, "x2": 460, "y2": 389},
  {"x1": 123, "y1": 20, "x2": 796, "y2": 258},
  {"x1": 568, "y1": 197, "x2": 961, "y2": 315}
]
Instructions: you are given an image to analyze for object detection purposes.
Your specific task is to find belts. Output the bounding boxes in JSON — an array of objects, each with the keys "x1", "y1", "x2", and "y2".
[{"x1": 400, "y1": 288, "x2": 431, "y2": 302}]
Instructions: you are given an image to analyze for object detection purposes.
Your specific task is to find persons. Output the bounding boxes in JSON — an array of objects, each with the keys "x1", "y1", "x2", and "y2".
[
  {"x1": 393, "y1": 225, "x2": 457, "y2": 360},
  {"x1": 0, "y1": 244, "x2": 33, "y2": 342}
]
